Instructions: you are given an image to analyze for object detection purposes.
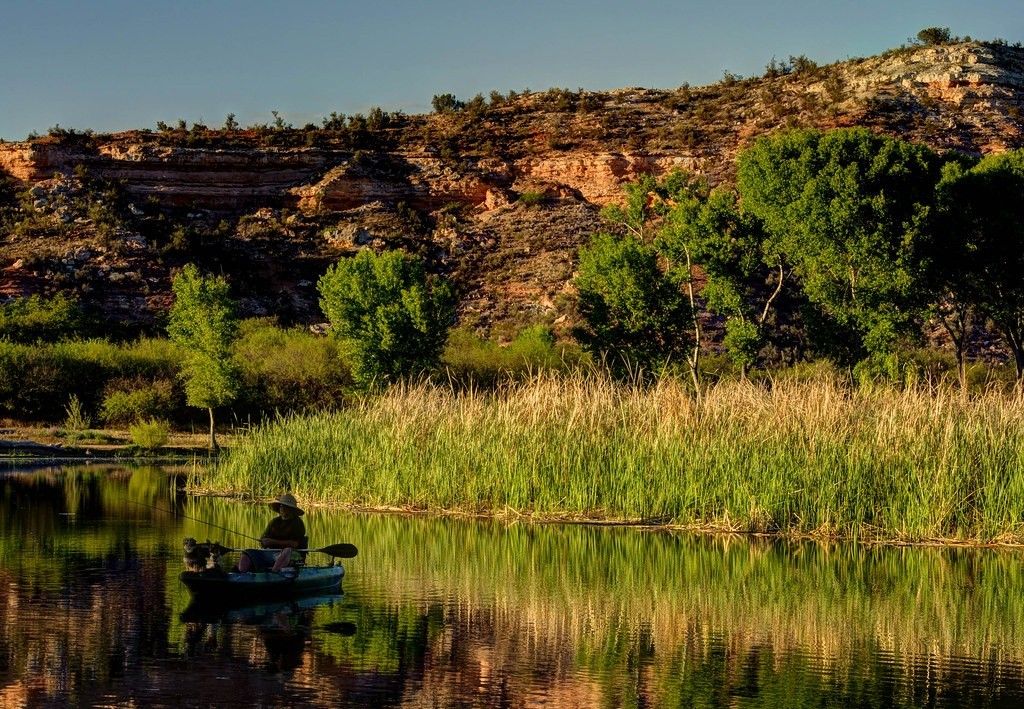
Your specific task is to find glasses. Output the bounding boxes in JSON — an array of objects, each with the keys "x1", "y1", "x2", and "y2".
[{"x1": 280, "y1": 504, "x2": 286, "y2": 507}]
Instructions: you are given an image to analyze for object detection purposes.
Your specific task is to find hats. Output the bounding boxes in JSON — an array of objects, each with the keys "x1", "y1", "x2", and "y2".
[{"x1": 268, "y1": 494, "x2": 304, "y2": 516}]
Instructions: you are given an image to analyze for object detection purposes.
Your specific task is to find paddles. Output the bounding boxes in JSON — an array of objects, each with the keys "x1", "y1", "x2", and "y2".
[{"x1": 196, "y1": 542, "x2": 358, "y2": 559}]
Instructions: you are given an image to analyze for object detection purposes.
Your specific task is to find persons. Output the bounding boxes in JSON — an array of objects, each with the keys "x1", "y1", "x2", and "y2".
[{"x1": 239, "y1": 494, "x2": 306, "y2": 571}]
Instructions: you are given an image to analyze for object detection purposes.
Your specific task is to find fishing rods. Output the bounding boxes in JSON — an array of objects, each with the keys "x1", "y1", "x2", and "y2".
[{"x1": 120, "y1": 497, "x2": 262, "y2": 541}]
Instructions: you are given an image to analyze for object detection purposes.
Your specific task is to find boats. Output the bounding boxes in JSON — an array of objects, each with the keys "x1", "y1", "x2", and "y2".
[{"x1": 178, "y1": 565, "x2": 345, "y2": 602}]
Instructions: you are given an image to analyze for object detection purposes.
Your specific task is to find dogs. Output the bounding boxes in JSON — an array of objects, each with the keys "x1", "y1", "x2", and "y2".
[{"x1": 183, "y1": 538, "x2": 225, "y2": 572}]
